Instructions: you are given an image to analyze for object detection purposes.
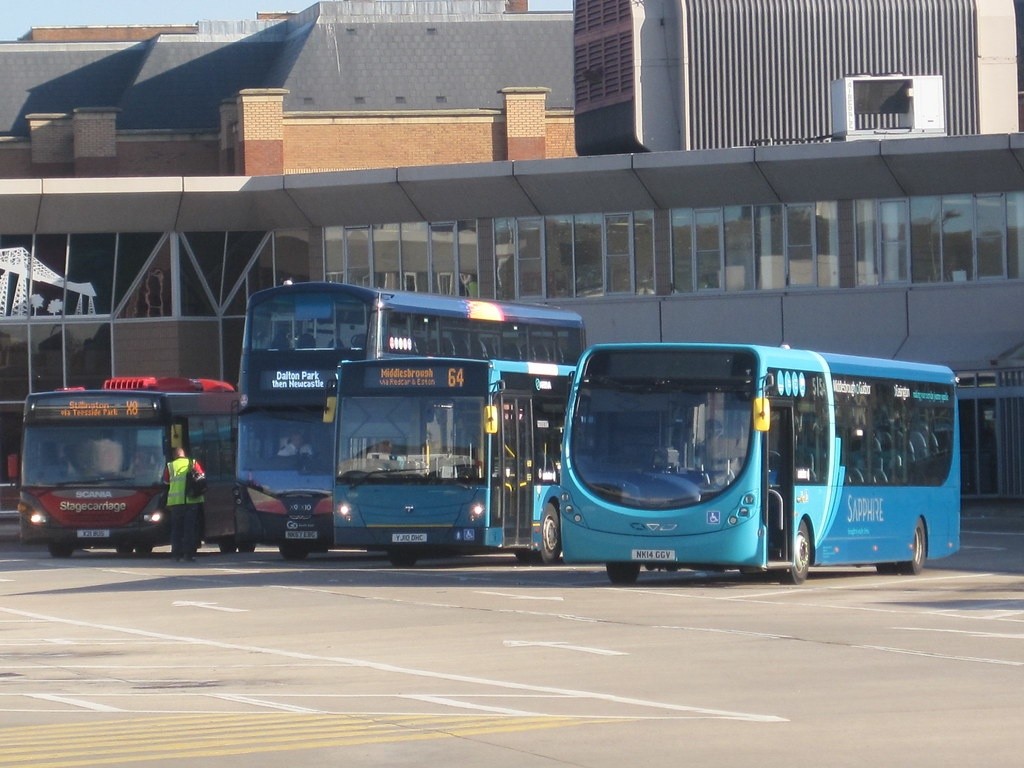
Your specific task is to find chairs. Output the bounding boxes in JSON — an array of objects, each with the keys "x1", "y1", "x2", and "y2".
[{"x1": 21, "y1": 320, "x2": 939, "y2": 485}]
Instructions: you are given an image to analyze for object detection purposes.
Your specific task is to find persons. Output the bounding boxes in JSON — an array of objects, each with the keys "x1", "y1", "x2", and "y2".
[
  {"x1": 162, "y1": 447, "x2": 206, "y2": 562},
  {"x1": 277, "y1": 433, "x2": 313, "y2": 458}
]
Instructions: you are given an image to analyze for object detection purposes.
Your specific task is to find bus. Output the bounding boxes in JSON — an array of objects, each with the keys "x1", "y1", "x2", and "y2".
[
  {"x1": 554, "y1": 340, "x2": 965, "y2": 586},
  {"x1": 6, "y1": 376, "x2": 257, "y2": 545},
  {"x1": 229, "y1": 281, "x2": 586, "y2": 566},
  {"x1": 334, "y1": 357, "x2": 580, "y2": 567}
]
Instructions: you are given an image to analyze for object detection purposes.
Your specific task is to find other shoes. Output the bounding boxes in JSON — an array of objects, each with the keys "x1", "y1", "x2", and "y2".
[{"x1": 172, "y1": 555, "x2": 196, "y2": 562}]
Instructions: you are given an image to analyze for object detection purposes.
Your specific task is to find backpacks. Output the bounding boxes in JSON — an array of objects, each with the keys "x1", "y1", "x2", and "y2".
[{"x1": 186, "y1": 458, "x2": 208, "y2": 497}]
